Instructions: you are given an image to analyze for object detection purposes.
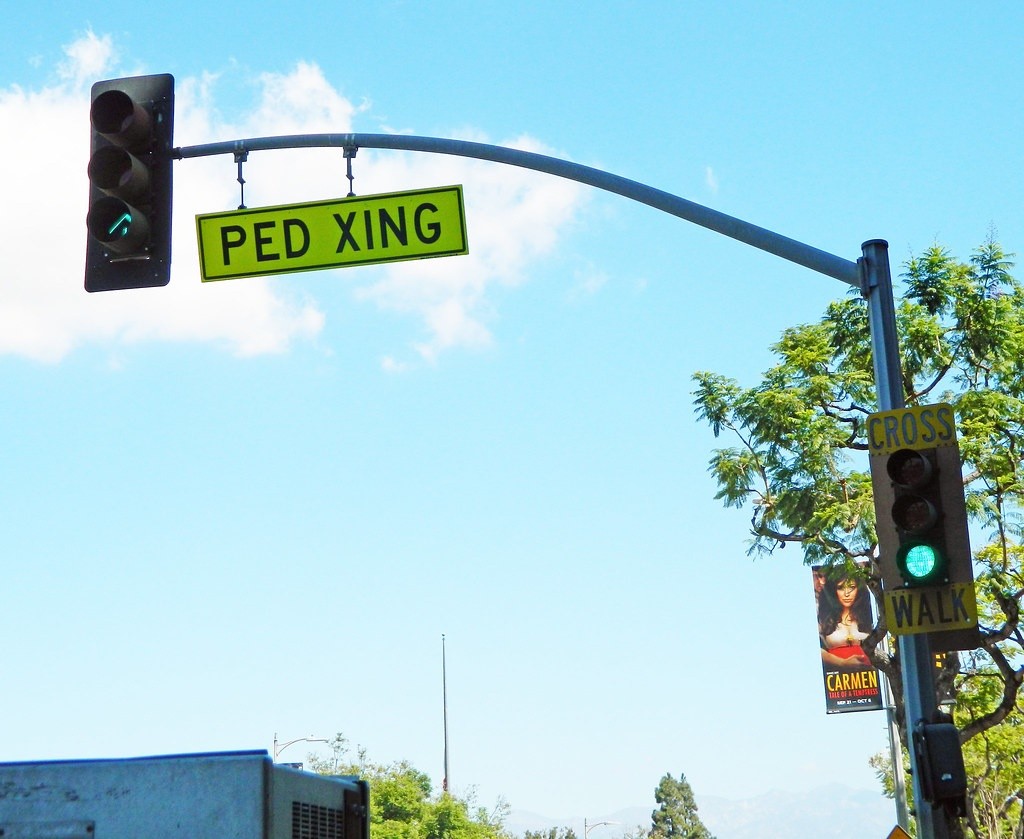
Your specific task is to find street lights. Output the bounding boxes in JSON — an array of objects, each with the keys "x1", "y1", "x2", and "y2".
[{"x1": 272, "y1": 730, "x2": 329, "y2": 763}]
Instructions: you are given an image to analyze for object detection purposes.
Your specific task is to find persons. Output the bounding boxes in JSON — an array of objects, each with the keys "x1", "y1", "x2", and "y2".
[{"x1": 812, "y1": 562, "x2": 873, "y2": 669}]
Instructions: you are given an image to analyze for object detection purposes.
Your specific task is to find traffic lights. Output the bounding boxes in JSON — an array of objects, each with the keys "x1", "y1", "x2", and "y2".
[
  {"x1": 869, "y1": 403, "x2": 981, "y2": 657},
  {"x1": 83, "y1": 72, "x2": 175, "y2": 292}
]
